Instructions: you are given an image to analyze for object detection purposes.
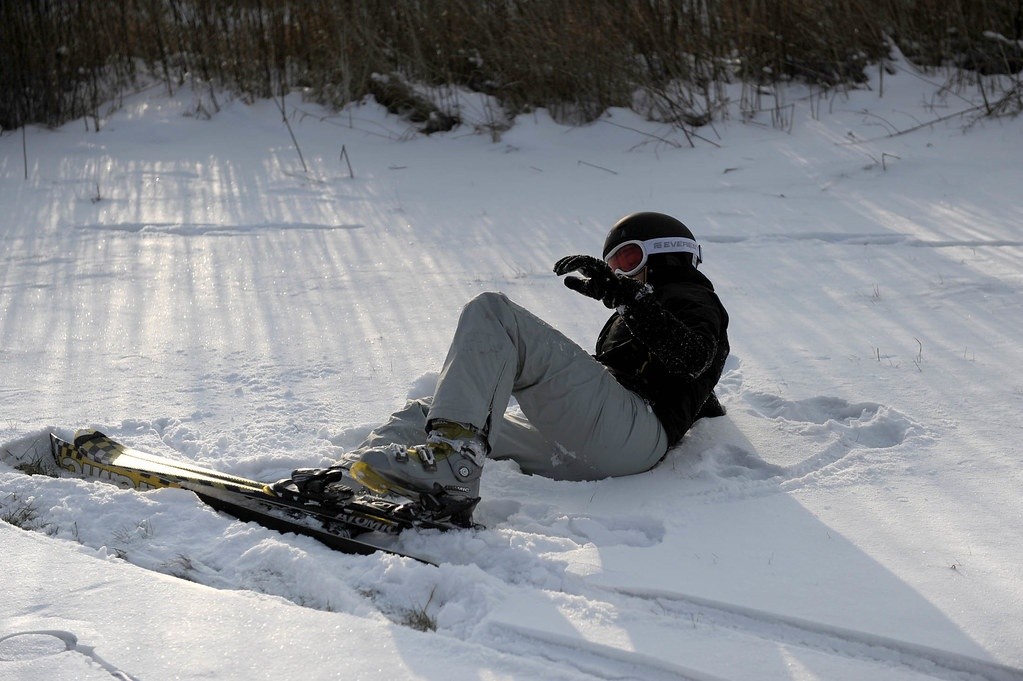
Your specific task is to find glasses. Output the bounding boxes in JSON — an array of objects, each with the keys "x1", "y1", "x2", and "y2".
[{"x1": 604, "y1": 240, "x2": 648, "y2": 277}]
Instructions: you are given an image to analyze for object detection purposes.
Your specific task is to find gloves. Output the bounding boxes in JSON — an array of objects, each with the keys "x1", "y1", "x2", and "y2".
[{"x1": 552, "y1": 254, "x2": 626, "y2": 310}]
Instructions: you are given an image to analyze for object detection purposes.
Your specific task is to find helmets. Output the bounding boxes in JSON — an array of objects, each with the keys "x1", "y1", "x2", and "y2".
[{"x1": 602, "y1": 212, "x2": 699, "y2": 287}]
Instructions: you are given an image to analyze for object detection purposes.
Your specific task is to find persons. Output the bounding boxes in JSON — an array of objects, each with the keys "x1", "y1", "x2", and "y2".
[{"x1": 263, "y1": 210, "x2": 731, "y2": 525}]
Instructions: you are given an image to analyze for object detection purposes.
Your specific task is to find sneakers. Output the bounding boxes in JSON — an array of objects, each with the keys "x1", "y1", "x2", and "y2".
[
  {"x1": 360, "y1": 419, "x2": 489, "y2": 501},
  {"x1": 271, "y1": 466, "x2": 372, "y2": 507}
]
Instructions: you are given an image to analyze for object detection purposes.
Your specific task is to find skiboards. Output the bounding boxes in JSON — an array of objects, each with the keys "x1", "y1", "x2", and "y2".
[{"x1": 48, "y1": 429, "x2": 463, "y2": 566}]
